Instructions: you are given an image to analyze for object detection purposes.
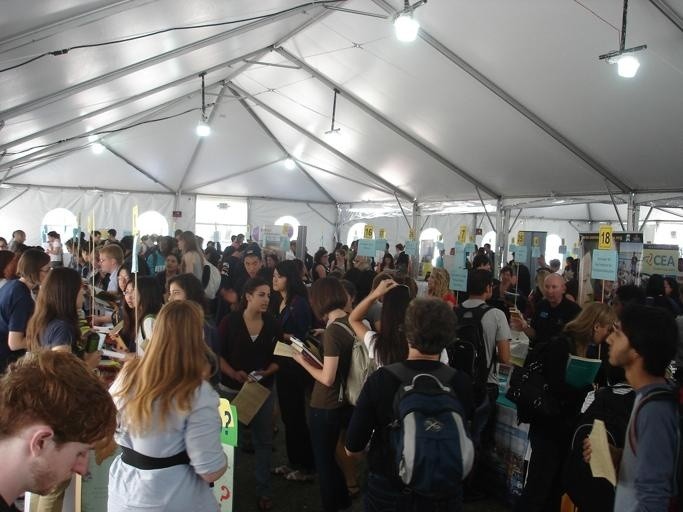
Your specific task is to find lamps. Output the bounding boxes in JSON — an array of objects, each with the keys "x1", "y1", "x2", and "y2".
[
  {"x1": 596, "y1": 1, "x2": 648, "y2": 81},
  {"x1": 391, "y1": 0, "x2": 424, "y2": 46},
  {"x1": 196, "y1": 71, "x2": 211, "y2": 138}
]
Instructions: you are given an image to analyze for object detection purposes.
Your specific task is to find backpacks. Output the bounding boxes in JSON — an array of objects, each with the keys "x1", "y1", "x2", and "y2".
[
  {"x1": 628, "y1": 387, "x2": 683, "y2": 511},
  {"x1": 379, "y1": 361, "x2": 478, "y2": 501},
  {"x1": 563, "y1": 386, "x2": 641, "y2": 512},
  {"x1": 199, "y1": 257, "x2": 222, "y2": 300},
  {"x1": 445, "y1": 301, "x2": 500, "y2": 383},
  {"x1": 331, "y1": 318, "x2": 374, "y2": 408}
]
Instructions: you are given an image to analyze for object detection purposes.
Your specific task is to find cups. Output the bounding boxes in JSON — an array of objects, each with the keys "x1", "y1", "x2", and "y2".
[{"x1": 83, "y1": 330, "x2": 99, "y2": 353}]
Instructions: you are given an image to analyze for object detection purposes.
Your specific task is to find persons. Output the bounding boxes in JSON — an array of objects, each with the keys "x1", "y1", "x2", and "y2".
[{"x1": 0, "y1": 225, "x2": 682, "y2": 512}]
